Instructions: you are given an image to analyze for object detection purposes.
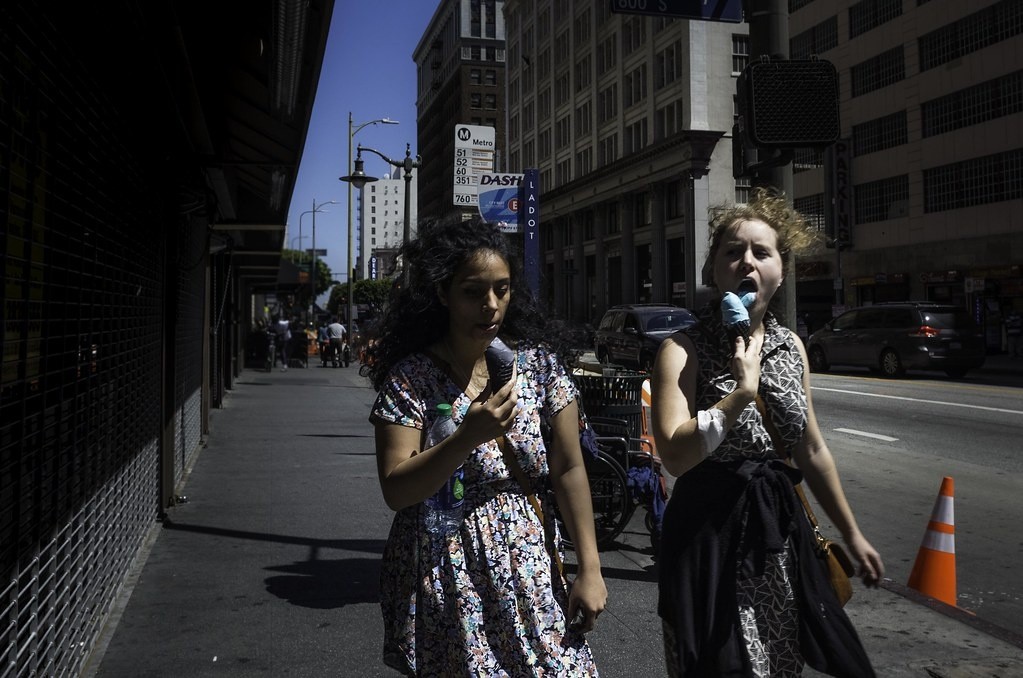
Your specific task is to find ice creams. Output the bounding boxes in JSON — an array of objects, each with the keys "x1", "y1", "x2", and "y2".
[
  {"x1": 721, "y1": 291, "x2": 750, "y2": 355},
  {"x1": 484, "y1": 337, "x2": 515, "y2": 402}
]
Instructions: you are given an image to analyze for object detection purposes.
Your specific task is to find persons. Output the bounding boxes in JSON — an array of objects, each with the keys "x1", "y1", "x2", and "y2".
[
  {"x1": 371, "y1": 221, "x2": 612, "y2": 678},
  {"x1": 270, "y1": 313, "x2": 361, "y2": 373},
  {"x1": 652, "y1": 195, "x2": 886, "y2": 678}
]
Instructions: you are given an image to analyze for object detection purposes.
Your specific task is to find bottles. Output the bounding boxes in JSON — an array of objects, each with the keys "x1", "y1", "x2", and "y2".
[{"x1": 422, "y1": 404, "x2": 467, "y2": 535}]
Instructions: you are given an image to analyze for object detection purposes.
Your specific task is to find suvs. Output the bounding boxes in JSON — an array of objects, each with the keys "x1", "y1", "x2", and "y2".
[
  {"x1": 594, "y1": 302, "x2": 701, "y2": 373},
  {"x1": 805, "y1": 300, "x2": 983, "y2": 381}
]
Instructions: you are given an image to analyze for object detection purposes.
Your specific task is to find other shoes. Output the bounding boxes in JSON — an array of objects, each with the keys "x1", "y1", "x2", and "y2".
[
  {"x1": 333, "y1": 363, "x2": 337, "y2": 368},
  {"x1": 339, "y1": 362, "x2": 343, "y2": 367}
]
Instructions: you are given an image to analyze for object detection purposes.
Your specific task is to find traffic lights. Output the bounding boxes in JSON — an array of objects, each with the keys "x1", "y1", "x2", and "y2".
[{"x1": 735, "y1": 52, "x2": 841, "y2": 156}]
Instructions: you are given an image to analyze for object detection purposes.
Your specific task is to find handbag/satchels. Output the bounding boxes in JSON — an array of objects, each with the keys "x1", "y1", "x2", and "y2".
[{"x1": 815, "y1": 537, "x2": 853, "y2": 610}]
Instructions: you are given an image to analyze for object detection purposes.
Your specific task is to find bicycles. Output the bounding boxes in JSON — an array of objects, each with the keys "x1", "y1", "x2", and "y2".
[{"x1": 263, "y1": 328, "x2": 392, "y2": 393}]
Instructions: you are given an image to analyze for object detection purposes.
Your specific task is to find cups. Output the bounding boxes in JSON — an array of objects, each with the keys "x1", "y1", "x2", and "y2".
[{"x1": 603, "y1": 369, "x2": 615, "y2": 388}]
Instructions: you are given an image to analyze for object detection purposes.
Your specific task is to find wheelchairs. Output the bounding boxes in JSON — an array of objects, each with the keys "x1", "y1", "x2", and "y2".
[{"x1": 555, "y1": 415, "x2": 667, "y2": 553}]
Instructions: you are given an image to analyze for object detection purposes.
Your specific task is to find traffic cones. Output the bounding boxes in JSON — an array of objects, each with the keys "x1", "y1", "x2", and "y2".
[{"x1": 904, "y1": 475, "x2": 956, "y2": 609}]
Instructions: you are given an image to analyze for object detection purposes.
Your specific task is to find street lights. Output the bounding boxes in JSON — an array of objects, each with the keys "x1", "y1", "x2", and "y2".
[
  {"x1": 311, "y1": 200, "x2": 337, "y2": 330},
  {"x1": 338, "y1": 140, "x2": 421, "y2": 288},
  {"x1": 289, "y1": 209, "x2": 330, "y2": 265},
  {"x1": 346, "y1": 111, "x2": 400, "y2": 348}
]
